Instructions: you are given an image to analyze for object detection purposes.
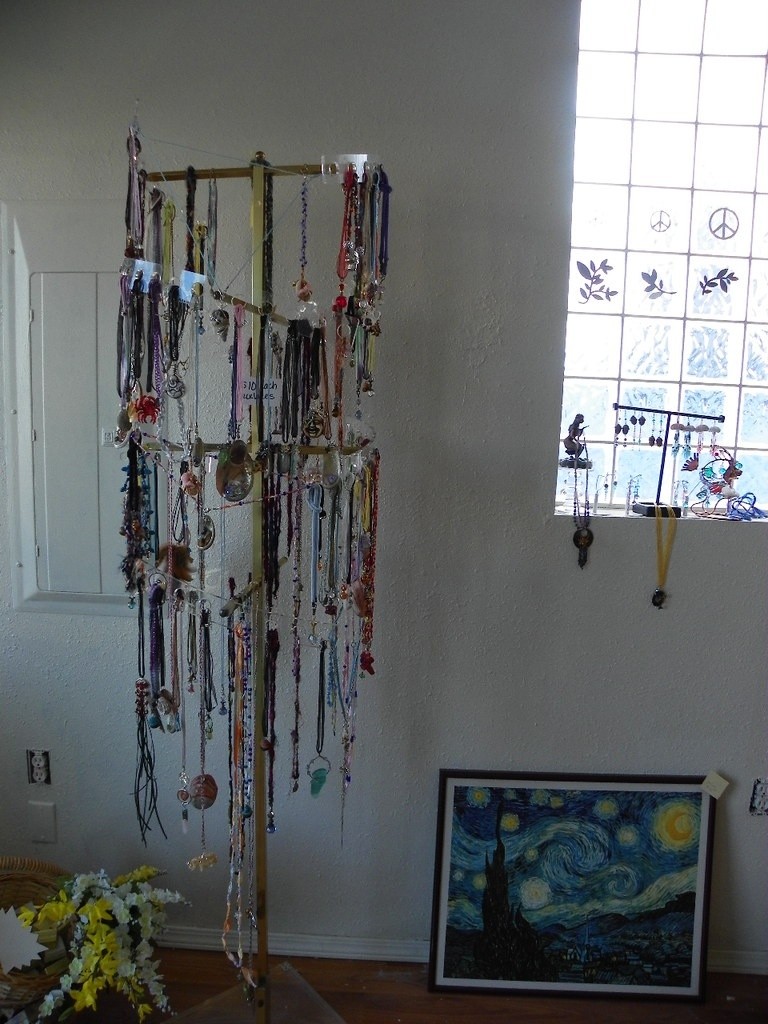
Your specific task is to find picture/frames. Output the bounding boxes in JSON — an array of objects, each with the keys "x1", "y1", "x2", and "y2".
[{"x1": 428, "y1": 767, "x2": 719, "y2": 1005}]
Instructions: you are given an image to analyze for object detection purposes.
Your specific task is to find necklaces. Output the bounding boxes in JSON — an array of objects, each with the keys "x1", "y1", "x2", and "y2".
[
  {"x1": 118, "y1": 130, "x2": 392, "y2": 1006},
  {"x1": 652, "y1": 502, "x2": 676, "y2": 608},
  {"x1": 574, "y1": 436, "x2": 594, "y2": 569}
]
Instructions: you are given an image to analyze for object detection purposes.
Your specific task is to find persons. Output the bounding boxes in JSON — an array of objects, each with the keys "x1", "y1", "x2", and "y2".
[{"x1": 564, "y1": 414, "x2": 589, "y2": 458}]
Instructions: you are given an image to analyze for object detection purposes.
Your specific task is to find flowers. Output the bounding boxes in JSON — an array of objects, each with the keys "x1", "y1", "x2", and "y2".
[{"x1": 17, "y1": 867, "x2": 192, "y2": 1024}]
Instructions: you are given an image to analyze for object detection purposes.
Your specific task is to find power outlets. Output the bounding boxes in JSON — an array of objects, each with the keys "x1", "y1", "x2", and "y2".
[
  {"x1": 748, "y1": 778, "x2": 768, "y2": 816},
  {"x1": 26, "y1": 749, "x2": 51, "y2": 786}
]
